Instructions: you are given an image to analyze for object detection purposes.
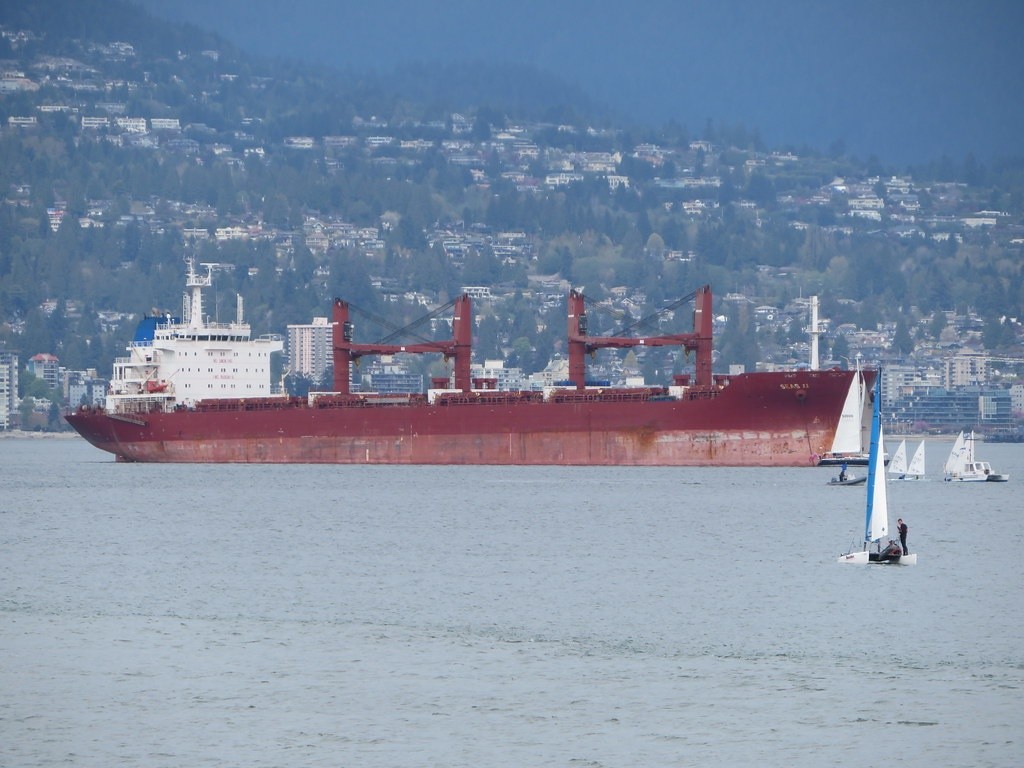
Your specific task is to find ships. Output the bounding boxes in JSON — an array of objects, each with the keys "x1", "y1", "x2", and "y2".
[{"x1": 63, "y1": 256, "x2": 883, "y2": 473}]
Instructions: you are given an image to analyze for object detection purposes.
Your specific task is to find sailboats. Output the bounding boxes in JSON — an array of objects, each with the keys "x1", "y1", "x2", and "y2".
[
  {"x1": 904, "y1": 440, "x2": 933, "y2": 482},
  {"x1": 943, "y1": 430, "x2": 1010, "y2": 483},
  {"x1": 885, "y1": 439, "x2": 913, "y2": 482},
  {"x1": 817, "y1": 357, "x2": 889, "y2": 468},
  {"x1": 837, "y1": 365, "x2": 919, "y2": 567}
]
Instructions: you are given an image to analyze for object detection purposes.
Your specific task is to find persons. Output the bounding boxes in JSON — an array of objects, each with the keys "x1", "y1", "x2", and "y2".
[
  {"x1": 839, "y1": 461, "x2": 848, "y2": 481},
  {"x1": 896, "y1": 519, "x2": 908, "y2": 555}
]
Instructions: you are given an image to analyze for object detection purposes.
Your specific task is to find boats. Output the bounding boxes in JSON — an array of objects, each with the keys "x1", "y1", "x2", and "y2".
[
  {"x1": 983, "y1": 433, "x2": 1024, "y2": 443},
  {"x1": 826, "y1": 461, "x2": 867, "y2": 487}
]
